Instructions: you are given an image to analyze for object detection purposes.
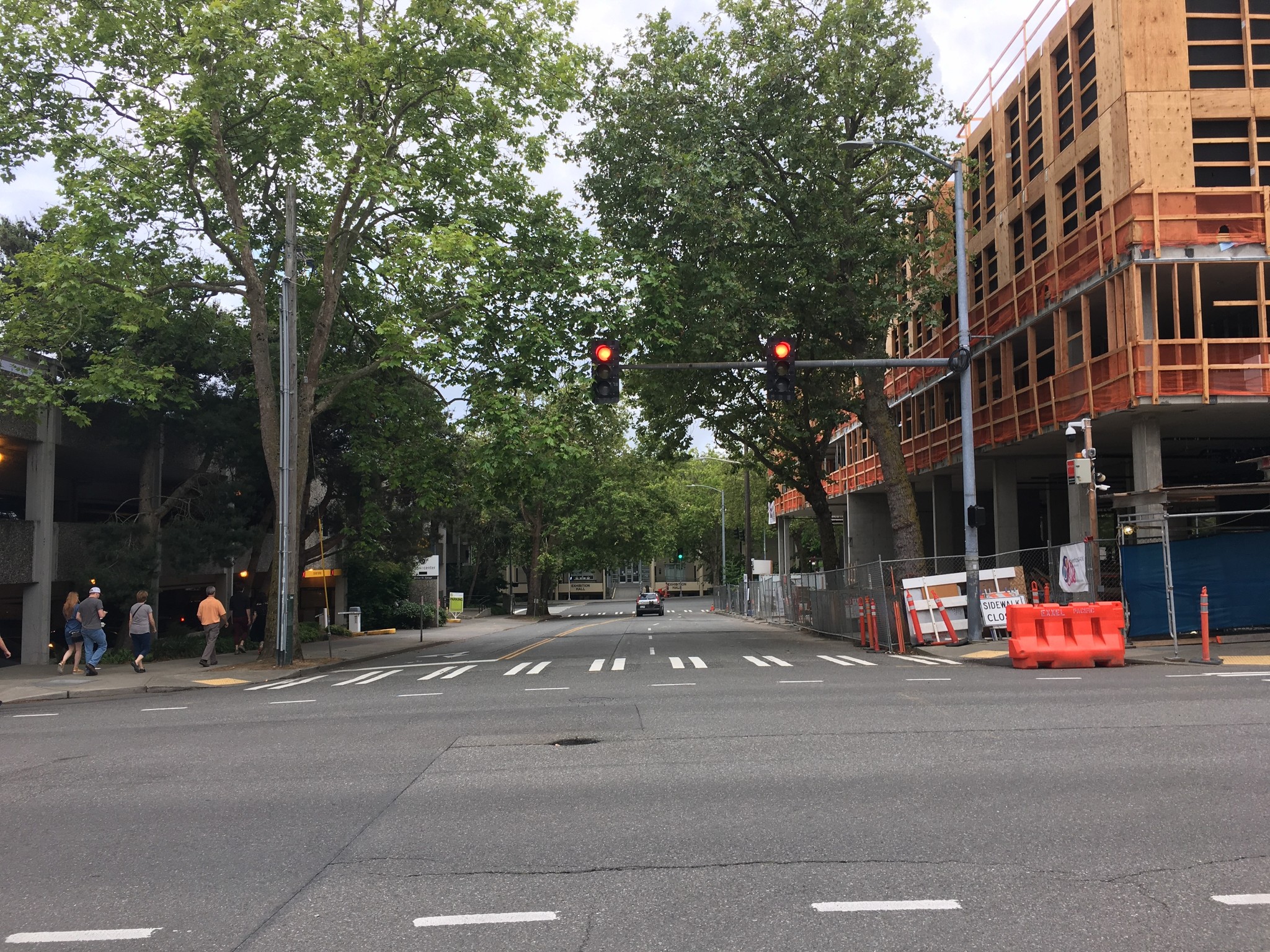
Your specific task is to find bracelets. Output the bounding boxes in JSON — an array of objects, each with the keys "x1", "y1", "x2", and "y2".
[{"x1": 248, "y1": 620, "x2": 251, "y2": 621}]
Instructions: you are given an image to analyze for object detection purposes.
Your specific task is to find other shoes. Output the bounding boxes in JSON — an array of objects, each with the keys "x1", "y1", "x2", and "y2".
[
  {"x1": 131, "y1": 661, "x2": 139, "y2": 672},
  {"x1": 58, "y1": 662, "x2": 64, "y2": 675},
  {"x1": 199, "y1": 660, "x2": 210, "y2": 667},
  {"x1": 140, "y1": 667, "x2": 146, "y2": 673},
  {"x1": 238, "y1": 644, "x2": 246, "y2": 653},
  {"x1": 235, "y1": 650, "x2": 240, "y2": 655},
  {"x1": 72, "y1": 668, "x2": 85, "y2": 675},
  {"x1": 85, "y1": 663, "x2": 98, "y2": 676},
  {"x1": 212, "y1": 661, "x2": 218, "y2": 665}
]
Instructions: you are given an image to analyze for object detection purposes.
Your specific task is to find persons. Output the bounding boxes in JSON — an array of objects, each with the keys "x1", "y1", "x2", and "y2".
[
  {"x1": 57, "y1": 591, "x2": 85, "y2": 675},
  {"x1": 250, "y1": 591, "x2": 269, "y2": 655},
  {"x1": 76, "y1": 587, "x2": 107, "y2": 676},
  {"x1": 662, "y1": 583, "x2": 669, "y2": 597},
  {"x1": 197, "y1": 586, "x2": 229, "y2": 667},
  {"x1": 229, "y1": 579, "x2": 251, "y2": 654},
  {"x1": 0, "y1": 635, "x2": 11, "y2": 658},
  {"x1": 129, "y1": 590, "x2": 157, "y2": 673}
]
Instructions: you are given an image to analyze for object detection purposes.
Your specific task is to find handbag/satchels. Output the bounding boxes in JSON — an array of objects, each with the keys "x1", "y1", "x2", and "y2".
[{"x1": 71, "y1": 630, "x2": 83, "y2": 643}]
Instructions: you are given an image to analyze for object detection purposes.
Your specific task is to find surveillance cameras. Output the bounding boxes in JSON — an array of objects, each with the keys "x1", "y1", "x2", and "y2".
[
  {"x1": 1099, "y1": 485, "x2": 1111, "y2": 491},
  {"x1": 1065, "y1": 427, "x2": 1076, "y2": 442}
]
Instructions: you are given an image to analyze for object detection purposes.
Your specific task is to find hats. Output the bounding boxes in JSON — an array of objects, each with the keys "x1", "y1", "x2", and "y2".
[{"x1": 89, "y1": 587, "x2": 103, "y2": 596}]
